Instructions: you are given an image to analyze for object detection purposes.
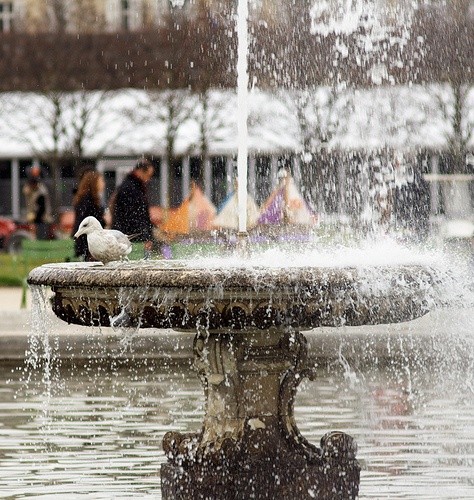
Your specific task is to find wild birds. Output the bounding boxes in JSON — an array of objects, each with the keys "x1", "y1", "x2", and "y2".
[{"x1": 74, "y1": 216, "x2": 143, "y2": 262}]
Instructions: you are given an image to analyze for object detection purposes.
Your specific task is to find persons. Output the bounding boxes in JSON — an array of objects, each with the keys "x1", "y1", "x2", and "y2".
[
  {"x1": 110, "y1": 162, "x2": 154, "y2": 260},
  {"x1": 73, "y1": 171, "x2": 107, "y2": 262},
  {"x1": 27, "y1": 176, "x2": 53, "y2": 239}
]
